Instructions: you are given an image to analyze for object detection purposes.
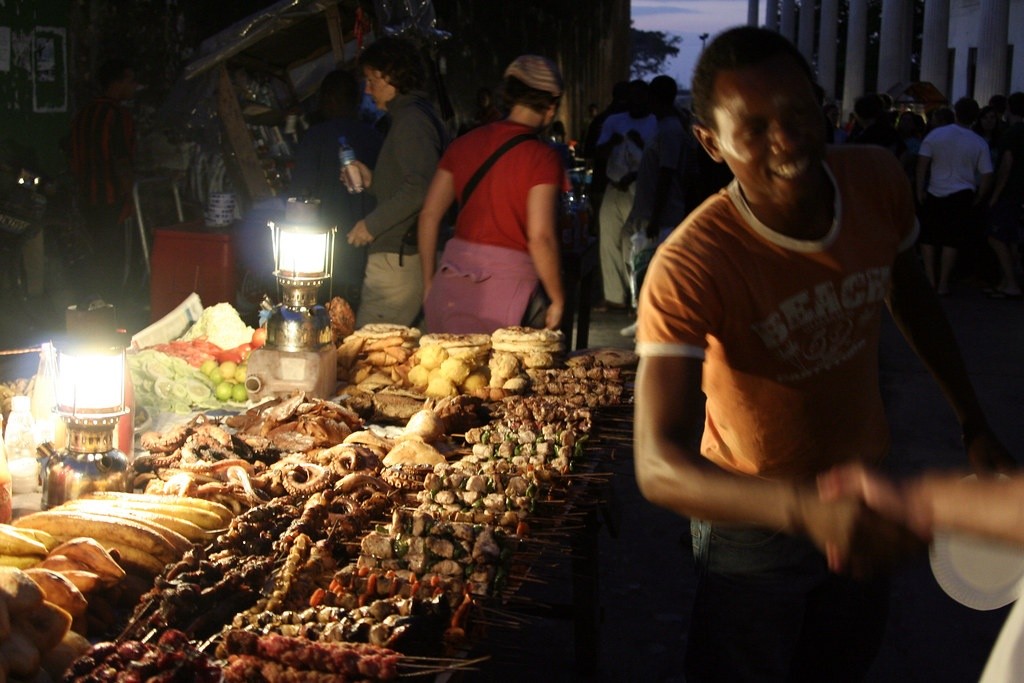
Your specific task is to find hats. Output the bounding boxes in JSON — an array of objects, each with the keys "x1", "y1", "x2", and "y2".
[{"x1": 504, "y1": 53, "x2": 563, "y2": 96}]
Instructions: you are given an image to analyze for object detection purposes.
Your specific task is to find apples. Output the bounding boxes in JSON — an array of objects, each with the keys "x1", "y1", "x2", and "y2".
[{"x1": 236, "y1": 328, "x2": 266, "y2": 363}]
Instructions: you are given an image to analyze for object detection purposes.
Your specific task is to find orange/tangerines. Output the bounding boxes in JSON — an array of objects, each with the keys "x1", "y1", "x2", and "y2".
[{"x1": 201, "y1": 360, "x2": 248, "y2": 402}]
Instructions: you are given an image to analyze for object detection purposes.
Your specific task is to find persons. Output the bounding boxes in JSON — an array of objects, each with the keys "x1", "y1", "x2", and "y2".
[
  {"x1": 0, "y1": 104, "x2": 57, "y2": 320},
  {"x1": 815, "y1": 456, "x2": 1024, "y2": 683},
  {"x1": 917, "y1": 96, "x2": 995, "y2": 300},
  {"x1": 284, "y1": 35, "x2": 574, "y2": 360},
  {"x1": 582, "y1": 23, "x2": 1024, "y2": 341},
  {"x1": 65, "y1": 60, "x2": 140, "y2": 352},
  {"x1": 630, "y1": 26, "x2": 1024, "y2": 682}
]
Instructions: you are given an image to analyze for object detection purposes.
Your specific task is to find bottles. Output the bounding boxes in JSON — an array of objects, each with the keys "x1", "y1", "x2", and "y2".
[
  {"x1": 239, "y1": 70, "x2": 310, "y2": 197},
  {"x1": 5, "y1": 396, "x2": 37, "y2": 495},
  {"x1": 0, "y1": 415, "x2": 12, "y2": 524},
  {"x1": 556, "y1": 190, "x2": 590, "y2": 247},
  {"x1": 337, "y1": 136, "x2": 364, "y2": 193}
]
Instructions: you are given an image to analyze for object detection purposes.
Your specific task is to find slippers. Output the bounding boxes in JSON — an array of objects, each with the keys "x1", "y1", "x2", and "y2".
[
  {"x1": 979, "y1": 287, "x2": 1024, "y2": 301},
  {"x1": 938, "y1": 288, "x2": 952, "y2": 297}
]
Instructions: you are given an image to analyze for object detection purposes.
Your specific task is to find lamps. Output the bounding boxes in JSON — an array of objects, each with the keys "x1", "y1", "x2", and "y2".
[
  {"x1": 36, "y1": 295, "x2": 132, "y2": 511},
  {"x1": 267, "y1": 193, "x2": 338, "y2": 352}
]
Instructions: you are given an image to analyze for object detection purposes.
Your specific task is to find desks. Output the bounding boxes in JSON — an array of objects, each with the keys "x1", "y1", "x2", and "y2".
[{"x1": 149, "y1": 218, "x2": 263, "y2": 324}]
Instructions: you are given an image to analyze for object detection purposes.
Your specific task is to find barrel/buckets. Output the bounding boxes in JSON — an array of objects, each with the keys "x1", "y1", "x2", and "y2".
[{"x1": 246, "y1": 345, "x2": 337, "y2": 405}]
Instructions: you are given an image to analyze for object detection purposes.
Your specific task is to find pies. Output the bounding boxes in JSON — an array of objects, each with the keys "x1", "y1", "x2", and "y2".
[
  {"x1": 0, "y1": 488, "x2": 236, "y2": 683},
  {"x1": 342, "y1": 322, "x2": 563, "y2": 370}
]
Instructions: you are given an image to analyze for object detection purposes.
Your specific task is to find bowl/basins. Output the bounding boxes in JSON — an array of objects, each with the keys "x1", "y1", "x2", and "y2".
[{"x1": 203, "y1": 192, "x2": 235, "y2": 226}]
